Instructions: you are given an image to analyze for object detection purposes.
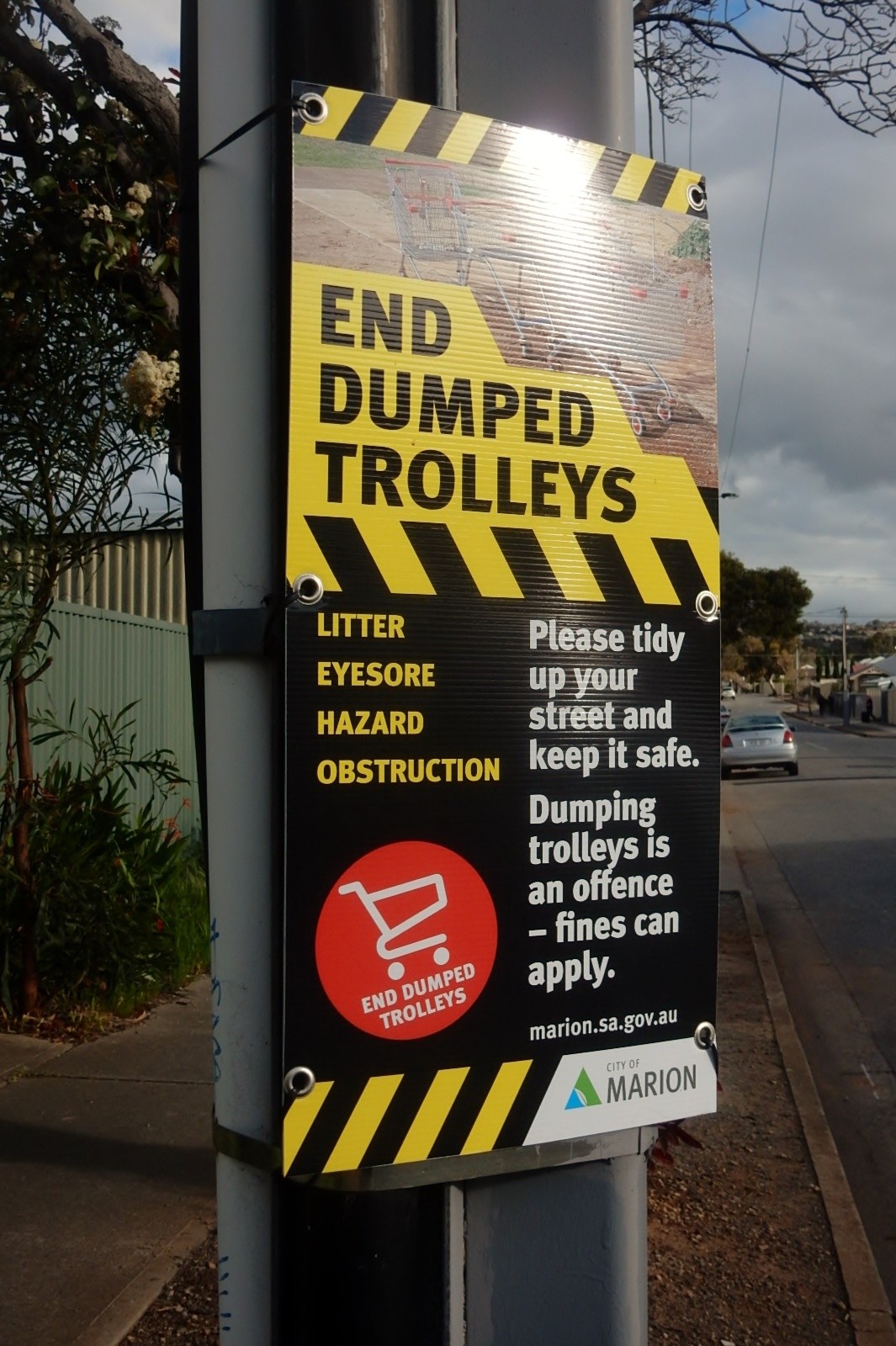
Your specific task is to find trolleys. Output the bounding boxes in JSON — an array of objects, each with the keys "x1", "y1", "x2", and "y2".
[{"x1": 383, "y1": 158, "x2": 689, "y2": 437}]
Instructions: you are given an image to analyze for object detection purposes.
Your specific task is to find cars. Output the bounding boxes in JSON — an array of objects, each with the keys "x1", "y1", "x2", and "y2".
[
  {"x1": 721, "y1": 687, "x2": 735, "y2": 700},
  {"x1": 720, "y1": 714, "x2": 799, "y2": 780},
  {"x1": 721, "y1": 705, "x2": 731, "y2": 724}
]
